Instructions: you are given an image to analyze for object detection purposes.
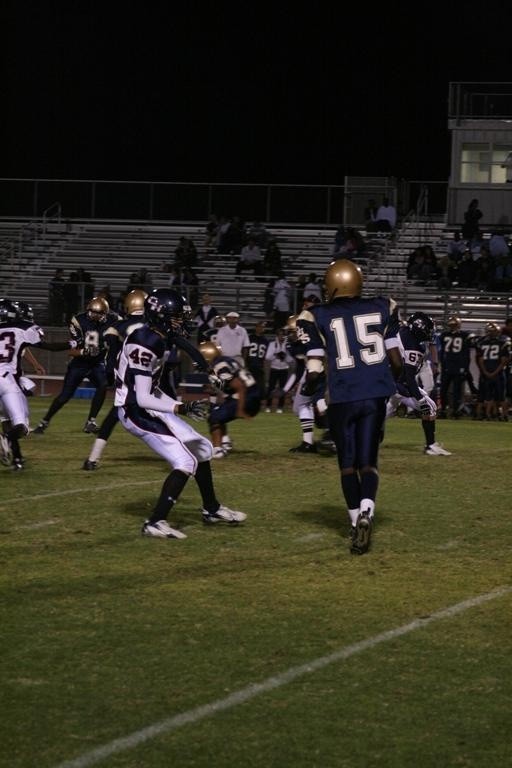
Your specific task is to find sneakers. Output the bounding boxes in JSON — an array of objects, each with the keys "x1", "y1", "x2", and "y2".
[
  {"x1": 13, "y1": 457, "x2": 25, "y2": 469},
  {"x1": 34, "y1": 418, "x2": 49, "y2": 432},
  {"x1": 275, "y1": 409, "x2": 283, "y2": 414},
  {"x1": 351, "y1": 511, "x2": 375, "y2": 555},
  {"x1": 83, "y1": 459, "x2": 97, "y2": 470},
  {"x1": 83, "y1": 417, "x2": 101, "y2": 434},
  {"x1": 0, "y1": 432, "x2": 12, "y2": 466},
  {"x1": 424, "y1": 441, "x2": 452, "y2": 456},
  {"x1": 141, "y1": 519, "x2": 188, "y2": 540},
  {"x1": 264, "y1": 408, "x2": 271, "y2": 413},
  {"x1": 435, "y1": 411, "x2": 512, "y2": 422},
  {"x1": 213, "y1": 443, "x2": 232, "y2": 459},
  {"x1": 289, "y1": 441, "x2": 317, "y2": 452},
  {"x1": 199, "y1": 504, "x2": 249, "y2": 524},
  {"x1": 349, "y1": 524, "x2": 356, "y2": 542}
]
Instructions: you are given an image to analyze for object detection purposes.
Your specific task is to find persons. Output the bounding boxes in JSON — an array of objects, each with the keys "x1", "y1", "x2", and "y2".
[
  {"x1": 112, "y1": 286, "x2": 248, "y2": 542},
  {"x1": 292, "y1": 257, "x2": 405, "y2": 555},
  {"x1": 1, "y1": 196, "x2": 511, "y2": 472}
]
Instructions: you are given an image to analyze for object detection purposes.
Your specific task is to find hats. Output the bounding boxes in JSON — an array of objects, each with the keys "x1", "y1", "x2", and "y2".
[
  {"x1": 304, "y1": 295, "x2": 320, "y2": 303},
  {"x1": 224, "y1": 312, "x2": 240, "y2": 318}
]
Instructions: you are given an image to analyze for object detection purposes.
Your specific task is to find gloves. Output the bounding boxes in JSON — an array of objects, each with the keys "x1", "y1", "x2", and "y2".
[
  {"x1": 420, "y1": 400, "x2": 432, "y2": 416},
  {"x1": 178, "y1": 398, "x2": 211, "y2": 423},
  {"x1": 300, "y1": 383, "x2": 316, "y2": 396},
  {"x1": 208, "y1": 369, "x2": 224, "y2": 394},
  {"x1": 83, "y1": 347, "x2": 100, "y2": 357},
  {"x1": 68, "y1": 335, "x2": 84, "y2": 350},
  {"x1": 275, "y1": 351, "x2": 286, "y2": 363}
]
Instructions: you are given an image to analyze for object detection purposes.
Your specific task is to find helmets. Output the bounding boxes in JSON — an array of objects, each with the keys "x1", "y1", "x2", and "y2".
[
  {"x1": 214, "y1": 315, "x2": 226, "y2": 327},
  {"x1": 446, "y1": 315, "x2": 461, "y2": 329},
  {"x1": 319, "y1": 258, "x2": 363, "y2": 304},
  {"x1": 192, "y1": 340, "x2": 223, "y2": 372},
  {"x1": 287, "y1": 315, "x2": 299, "y2": 342},
  {"x1": 484, "y1": 322, "x2": 502, "y2": 339},
  {"x1": 86, "y1": 297, "x2": 109, "y2": 323},
  {"x1": 122, "y1": 289, "x2": 149, "y2": 320},
  {"x1": 14, "y1": 301, "x2": 34, "y2": 324},
  {"x1": 144, "y1": 288, "x2": 192, "y2": 337},
  {"x1": 408, "y1": 312, "x2": 434, "y2": 341},
  {"x1": 0, "y1": 298, "x2": 18, "y2": 324}
]
